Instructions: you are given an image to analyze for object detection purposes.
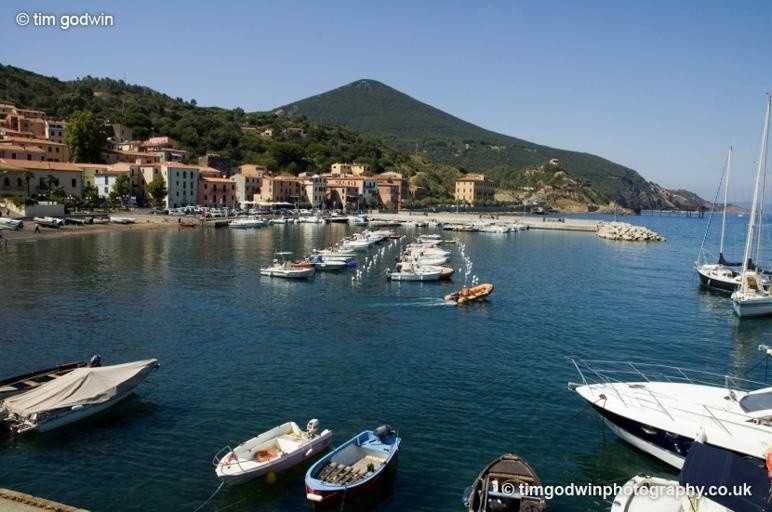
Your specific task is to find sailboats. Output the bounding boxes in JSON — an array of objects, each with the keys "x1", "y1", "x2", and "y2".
[
  {"x1": 730, "y1": 95, "x2": 772, "y2": 318},
  {"x1": 694, "y1": 146, "x2": 772, "y2": 293}
]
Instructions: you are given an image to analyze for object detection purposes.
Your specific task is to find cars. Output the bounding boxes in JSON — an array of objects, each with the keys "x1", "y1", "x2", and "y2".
[{"x1": 149, "y1": 205, "x2": 345, "y2": 216}]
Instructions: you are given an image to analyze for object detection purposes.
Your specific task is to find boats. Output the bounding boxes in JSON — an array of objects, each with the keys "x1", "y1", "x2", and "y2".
[
  {"x1": 564, "y1": 344, "x2": 772, "y2": 478},
  {"x1": 229, "y1": 215, "x2": 528, "y2": 281},
  {"x1": 0, "y1": 354, "x2": 102, "y2": 400},
  {"x1": 445, "y1": 283, "x2": 494, "y2": 303},
  {"x1": 33, "y1": 216, "x2": 137, "y2": 228},
  {"x1": 462, "y1": 453, "x2": 547, "y2": 512},
  {"x1": 212, "y1": 419, "x2": 333, "y2": 486},
  {"x1": 0, "y1": 358, "x2": 160, "y2": 434},
  {"x1": 0, "y1": 217, "x2": 23, "y2": 230},
  {"x1": 609, "y1": 472, "x2": 735, "y2": 512},
  {"x1": 305, "y1": 425, "x2": 402, "y2": 503}
]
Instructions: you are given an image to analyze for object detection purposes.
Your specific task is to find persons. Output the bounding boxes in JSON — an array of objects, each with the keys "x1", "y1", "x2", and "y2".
[{"x1": 462, "y1": 284, "x2": 469, "y2": 296}]
